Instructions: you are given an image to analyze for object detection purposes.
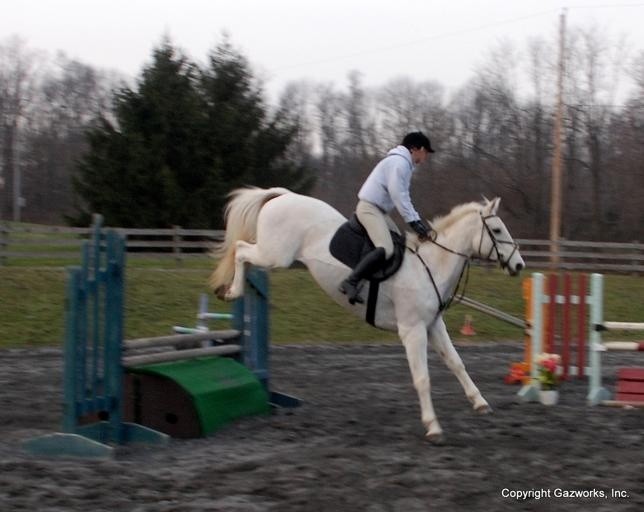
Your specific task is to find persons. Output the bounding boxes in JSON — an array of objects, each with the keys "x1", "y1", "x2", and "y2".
[{"x1": 338, "y1": 131, "x2": 436, "y2": 304}]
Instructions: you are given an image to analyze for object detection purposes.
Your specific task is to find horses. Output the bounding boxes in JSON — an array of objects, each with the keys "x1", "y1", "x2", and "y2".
[{"x1": 208, "y1": 182, "x2": 527, "y2": 447}]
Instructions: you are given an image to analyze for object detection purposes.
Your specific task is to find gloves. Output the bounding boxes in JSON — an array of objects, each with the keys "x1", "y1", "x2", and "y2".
[{"x1": 409, "y1": 220, "x2": 427, "y2": 236}]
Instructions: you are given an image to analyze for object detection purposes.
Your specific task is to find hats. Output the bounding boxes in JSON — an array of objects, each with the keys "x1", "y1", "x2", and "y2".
[{"x1": 402, "y1": 132, "x2": 435, "y2": 153}]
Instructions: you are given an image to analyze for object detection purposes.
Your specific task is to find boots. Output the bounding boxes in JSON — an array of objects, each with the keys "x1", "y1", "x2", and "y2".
[{"x1": 340, "y1": 247, "x2": 385, "y2": 306}]
